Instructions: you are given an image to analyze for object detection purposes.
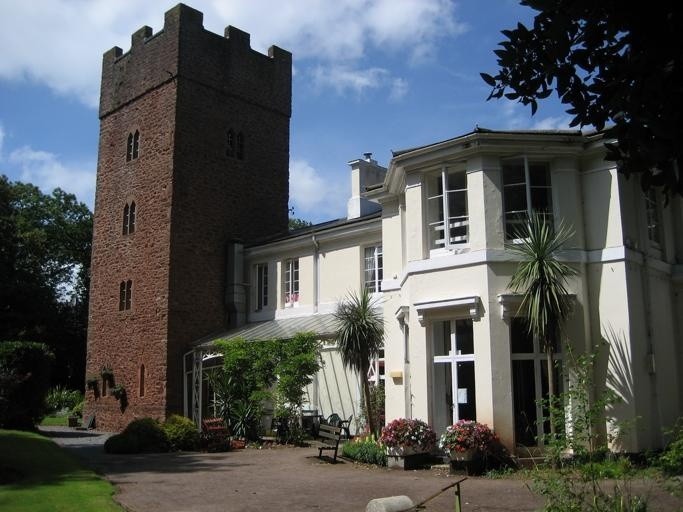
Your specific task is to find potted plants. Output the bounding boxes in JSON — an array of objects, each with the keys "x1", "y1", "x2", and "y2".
[
  {"x1": 228, "y1": 402, "x2": 258, "y2": 450},
  {"x1": 85, "y1": 363, "x2": 123, "y2": 400}
]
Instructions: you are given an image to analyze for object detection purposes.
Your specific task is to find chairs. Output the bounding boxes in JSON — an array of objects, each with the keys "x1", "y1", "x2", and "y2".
[{"x1": 318, "y1": 413, "x2": 353, "y2": 439}]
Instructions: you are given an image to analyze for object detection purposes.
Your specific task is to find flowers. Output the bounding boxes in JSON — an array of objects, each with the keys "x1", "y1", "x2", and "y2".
[{"x1": 375, "y1": 416, "x2": 499, "y2": 456}]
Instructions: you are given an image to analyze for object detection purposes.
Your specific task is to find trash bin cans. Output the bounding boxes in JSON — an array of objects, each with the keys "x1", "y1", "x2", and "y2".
[{"x1": 67, "y1": 417, "x2": 77, "y2": 427}]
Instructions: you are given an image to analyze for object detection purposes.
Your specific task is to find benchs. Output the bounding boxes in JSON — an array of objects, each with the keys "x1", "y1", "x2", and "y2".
[{"x1": 302, "y1": 424, "x2": 343, "y2": 465}]
